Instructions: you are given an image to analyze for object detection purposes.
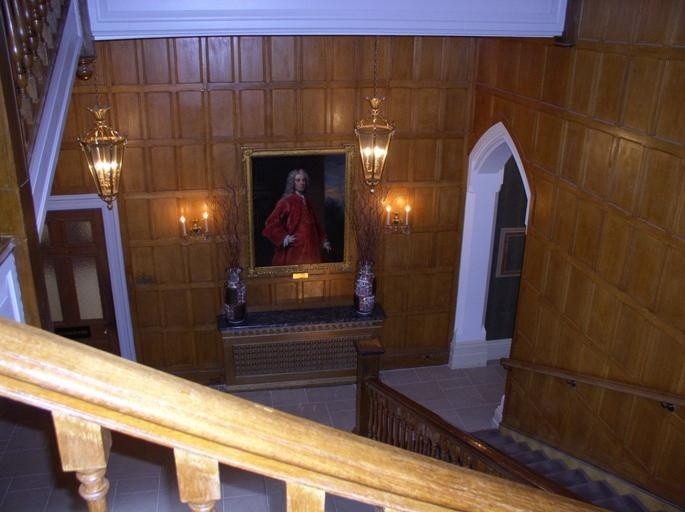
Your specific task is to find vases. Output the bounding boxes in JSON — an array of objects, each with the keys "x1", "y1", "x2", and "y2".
[
  {"x1": 353, "y1": 259, "x2": 376, "y2": 314},
  {"x1": 223, "y1": 267, "x2": 247, "y2": 323}
]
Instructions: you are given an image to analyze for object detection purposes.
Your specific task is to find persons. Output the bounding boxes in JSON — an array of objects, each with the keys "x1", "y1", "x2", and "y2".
[{"x1": 259, "y1": 168, "x2": 335, "y2": 266}]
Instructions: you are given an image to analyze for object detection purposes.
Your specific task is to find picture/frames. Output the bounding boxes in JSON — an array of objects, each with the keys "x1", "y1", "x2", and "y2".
[
  {"x1": 496, "y1": 227, "x2": 525, "y2": 277},
  {"x1": 240, "y1": 144, "x2": 352, "y2": 279}
]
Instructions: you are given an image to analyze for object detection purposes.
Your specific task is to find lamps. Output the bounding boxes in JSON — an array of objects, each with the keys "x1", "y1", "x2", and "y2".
[
  {"x1": 75, "y1": 61, "x2": 128, "y2": 210},
  {"x1": 354, "y1": 35, "x2": 395, "y2": 193},
  {"x1": 384, "y1": 205, "x2": 411, "y2": 234},
  {"x1": 179, "y1": 211, "x2": 210, "y2": 241}
]
